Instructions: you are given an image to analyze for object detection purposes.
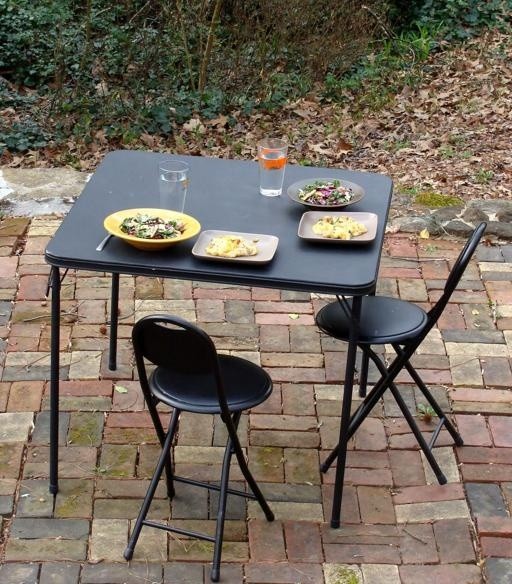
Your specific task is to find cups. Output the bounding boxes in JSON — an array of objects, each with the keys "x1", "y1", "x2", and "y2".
[
  {"x1": 159, "y1": 158, "x2": 190, "y2": 214},
  {"x1": 257, "y1": 138, "x2": 288, "y2": 197}
]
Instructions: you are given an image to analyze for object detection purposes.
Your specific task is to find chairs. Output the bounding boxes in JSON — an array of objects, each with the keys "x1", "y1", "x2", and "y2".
[
  {"x1": 123, "y1": 313, "x2": 275, "y2": 582},
  {"x1": 313, "y1": 221, "x2": 489, "y2": 486}
]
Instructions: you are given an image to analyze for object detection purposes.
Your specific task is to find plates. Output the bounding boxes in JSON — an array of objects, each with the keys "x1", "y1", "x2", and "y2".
[
  {"x1": 191, "y1": 230, "x2": 279, "y2": 264},
  {"x1": 287, "y1": 177, "x2": 365, "y2": 209},
  {"x1": 297, "y1": 210, "x2": 378, "y2": 244},
  {"x1": 104, "y1": 208, "x2": 201, "y2": 250}
]
